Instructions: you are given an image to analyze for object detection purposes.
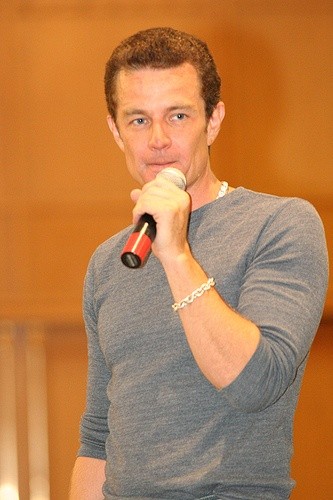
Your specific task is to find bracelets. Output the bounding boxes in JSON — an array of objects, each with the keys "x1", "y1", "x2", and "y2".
[{"x1": 172, "y1": 277, "x2": 217, "y2": 310}]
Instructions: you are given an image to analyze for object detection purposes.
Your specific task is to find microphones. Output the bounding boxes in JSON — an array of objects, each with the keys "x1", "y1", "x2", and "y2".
[{"x1": 120, "y1": 167, "x2": 186, "y2": 269}]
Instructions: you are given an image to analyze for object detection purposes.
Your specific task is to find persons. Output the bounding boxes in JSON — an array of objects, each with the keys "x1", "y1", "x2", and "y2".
[{"x1": 65, "y1": 27, "x2": 327, "y2": 499}]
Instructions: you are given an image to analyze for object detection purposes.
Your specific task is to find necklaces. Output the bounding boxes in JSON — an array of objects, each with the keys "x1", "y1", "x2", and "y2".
[{"x1": 217, "y1": 175, "x2": 233, "y2": 198}]
